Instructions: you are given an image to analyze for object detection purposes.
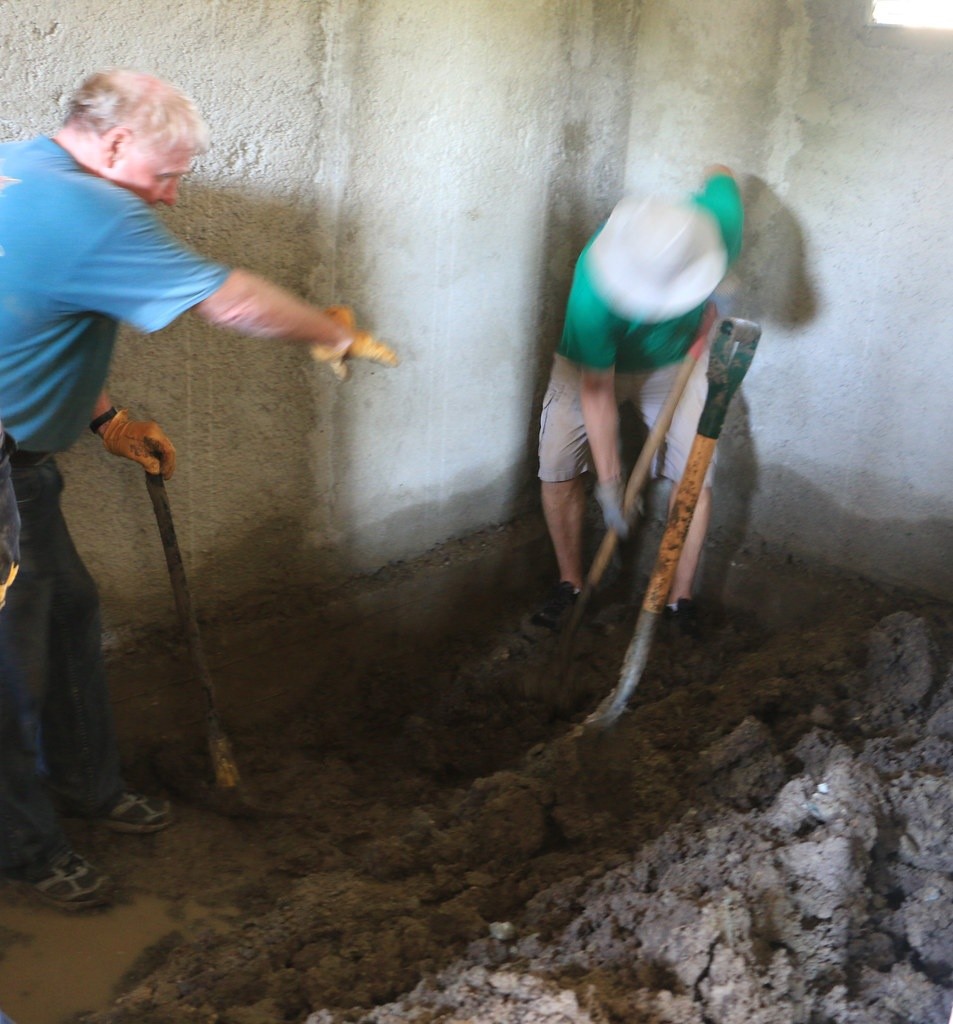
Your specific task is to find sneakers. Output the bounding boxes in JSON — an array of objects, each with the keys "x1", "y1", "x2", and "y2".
[
  {"x1": 662, "y1": 597, "x2": 697, "y2": 636},
  {"x1": 73, "y1": 789, "x2": 176, "y2": 833},
  {"x1": 532, "y1": 582, "x2": 581, "y2": 627},
  {"x1": 7, "y1": 842, "x2": 114, "y2": 911}
]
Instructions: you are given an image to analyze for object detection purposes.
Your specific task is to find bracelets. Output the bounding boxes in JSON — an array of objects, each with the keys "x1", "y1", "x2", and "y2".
[{"x1": 89, "y1": 402, "x2": 120, "y2": 435}]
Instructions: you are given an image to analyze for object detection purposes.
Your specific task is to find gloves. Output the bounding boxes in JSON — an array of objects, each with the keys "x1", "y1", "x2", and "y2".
[
  {"x1": 703, "y1": 270, "x2": 742, "y2": 318},
  {"x1": 595, "y1": 479, "x2": 643, "y2": 539},
  {"x1": 310, "y1": 305, "x2": 398, "y2": 381},
  {"x1": 103, "y1": 408, "x2": 176, "y2": 481}
]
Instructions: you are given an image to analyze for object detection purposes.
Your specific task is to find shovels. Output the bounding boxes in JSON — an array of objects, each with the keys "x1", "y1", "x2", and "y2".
[
  {"x1": 524, "y1": 300, "x2": 716, "y2": 714},
  {"x1": 545, "y1": 315, "x2": 766, "y2": 756},
  {"x1": 146, "y1": 469, "x2": 271, "y2": 819}
]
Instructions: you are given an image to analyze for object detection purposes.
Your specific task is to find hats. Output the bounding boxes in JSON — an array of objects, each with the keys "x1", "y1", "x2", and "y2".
[{"x1": 590, "y1": 191, "x2": 726, "y2": 323}]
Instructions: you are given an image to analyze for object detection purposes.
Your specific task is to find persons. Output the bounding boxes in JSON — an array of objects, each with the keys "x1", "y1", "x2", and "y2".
[
  {"x1": 0, "y1": 63, "x2": 403, "y2": 913},
  {"x1": 534, "y1": 163, "x2": 745, "y2": 648}
]
